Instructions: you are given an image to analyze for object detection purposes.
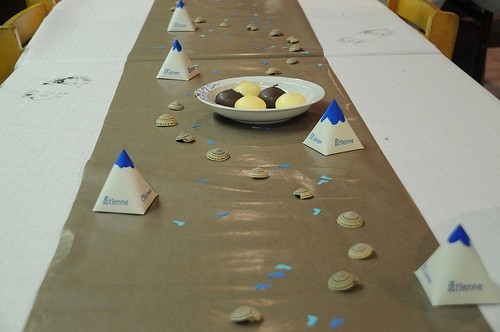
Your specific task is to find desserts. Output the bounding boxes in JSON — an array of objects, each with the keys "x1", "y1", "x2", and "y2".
[{"x1": 214, "y1": 82, "x2": 307, "y2": 110}]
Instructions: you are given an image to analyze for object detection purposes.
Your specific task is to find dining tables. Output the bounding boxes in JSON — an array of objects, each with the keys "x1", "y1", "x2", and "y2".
[{"x1": 0, "y1": 1, "x2": 499, "y2": 331}]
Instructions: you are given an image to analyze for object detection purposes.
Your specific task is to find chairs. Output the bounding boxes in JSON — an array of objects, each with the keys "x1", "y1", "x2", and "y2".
[
  {"x1": 387, "y1": 0, "x2": 459, "y2": 62},
  {"x1": 0, "y1": 26, "x2": 23, "y2": 86},
  {"x1": 3, "y1": 3, "x2": 48, "y2": 46},
  {"x1": 26, "y1": 0, "x2": 56, "y2": 11}
]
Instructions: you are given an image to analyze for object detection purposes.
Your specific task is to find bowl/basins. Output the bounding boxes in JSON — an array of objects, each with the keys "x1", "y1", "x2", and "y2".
[{"x1": 194, "y1": 75, "x2": 326, "y2": 124}]
